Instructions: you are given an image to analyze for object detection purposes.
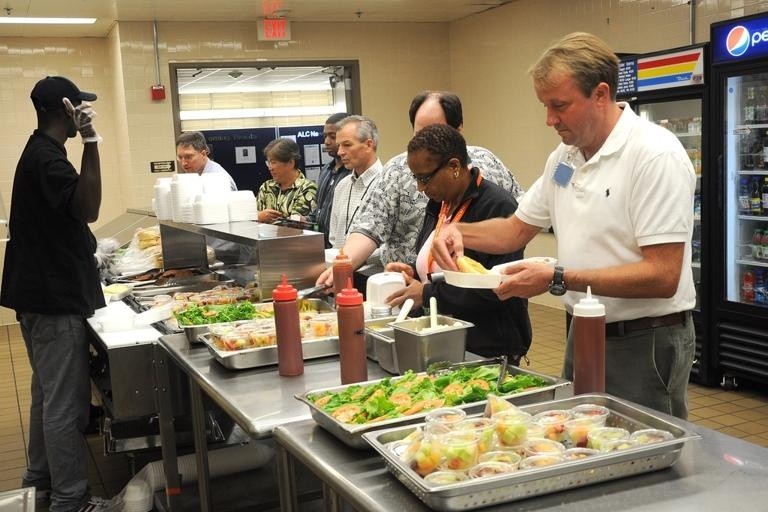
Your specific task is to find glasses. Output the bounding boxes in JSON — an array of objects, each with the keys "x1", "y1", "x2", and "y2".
[{"x1": 413, "y1": 163, "x2": 443, "y2": 184}]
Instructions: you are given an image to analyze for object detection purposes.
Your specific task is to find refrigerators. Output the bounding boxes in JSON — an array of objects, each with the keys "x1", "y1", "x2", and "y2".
[
  {"x1": 613, "y1": 41, "x2": 717, "y2": 389},
  {"x1": 708, "y1": 10, "x2": 768, "y2": 393}
]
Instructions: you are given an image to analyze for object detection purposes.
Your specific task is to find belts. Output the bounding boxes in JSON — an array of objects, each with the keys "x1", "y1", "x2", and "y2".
[{"x1": 604, "y1": 312, "x2": 684, "y2": 337}]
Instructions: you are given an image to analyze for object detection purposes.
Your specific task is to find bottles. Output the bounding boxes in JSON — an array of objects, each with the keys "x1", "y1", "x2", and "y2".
[
  {"x1": 573, "y1": 285, "x2": 607, "y2": 396},
  {"x1": 332, "y1": 247, "x2": 353, "y2": 309},
  {"x1": 335, "y1": 278, "x2": 368, "y2": 385},
  {"x1": 370, "y1": 305, "x2": 393, "y2": 319},
  {"x1": 657, "y1": 114, "x2": 702, "y2": 312},
  {"x1": 272, "y1": 273, "x2": 304, "y2": 377},
  {"x1": 738, "y1": 84, "x2": 768, "y2": 305}
]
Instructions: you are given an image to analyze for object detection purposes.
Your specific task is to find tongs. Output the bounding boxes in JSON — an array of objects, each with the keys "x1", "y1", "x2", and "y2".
[
  {"x1": 133, "y1": 278, "x2": 166, "y2": 290},
  {"x1": 111, "y1": 268, "x2": 162, "y2": 283},
  {"x1": 449, "y1": 354, "x2": 508, "y2": 392},
  {"x1": 296, "y1": 284, "x2": 328, "y2": 301}
]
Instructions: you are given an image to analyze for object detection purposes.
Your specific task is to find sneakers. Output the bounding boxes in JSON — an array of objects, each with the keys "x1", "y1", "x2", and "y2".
[{"x1": 77, "y1": 496, "x2": 111, "y2": 512}]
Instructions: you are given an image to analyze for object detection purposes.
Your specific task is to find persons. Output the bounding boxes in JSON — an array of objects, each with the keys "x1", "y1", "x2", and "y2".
[
  {"x1": 256, "y1": 135, "x2": 319, "y2": 226},
  {"x1": 315, "y1": 90, "x2": 526, "y2": 296},
  {"x1": 283, "y1": 111, "x2": 350, "y2": 248},
  {"x1": 174, "y1": 129, "x2": 239, "y2": 193},
  {"x1": 327, "y1": 115, "x2": 387, "y2": 304},
  {"x1": 428, "y1": 30, "x2": 697, "y2": 423},
  {"x1": 1, "y1": 72, "x2": 126, "y2": 512},
  {"x1": 380, "y1": 122, "x2": 534, "y2": 368}
]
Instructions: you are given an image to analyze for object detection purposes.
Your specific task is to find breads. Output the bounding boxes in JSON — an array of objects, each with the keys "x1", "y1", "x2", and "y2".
[{"x1": 136, "y1": 229, "x2": 164, "y2": 268}]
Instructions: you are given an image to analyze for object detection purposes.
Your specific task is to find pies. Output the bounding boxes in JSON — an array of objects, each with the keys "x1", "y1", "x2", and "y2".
[
  {"x1": 316, "y1": 375, "x2": 488, "y2": 426},
  {"x1": 456, "y1": 255, "x2": 490, "y2": 275}
]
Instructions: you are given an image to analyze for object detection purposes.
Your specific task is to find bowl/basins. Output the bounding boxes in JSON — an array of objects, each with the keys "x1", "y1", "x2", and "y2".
[
  {"x1": 206, "y1": 311, "x2": 341, "y2": 351},
  {"x1": 153, "y1": 172, "x2": 258, "y2": 225},
  {"x1": 388, "y1": 405, "x2": 677, "y2": 485}
]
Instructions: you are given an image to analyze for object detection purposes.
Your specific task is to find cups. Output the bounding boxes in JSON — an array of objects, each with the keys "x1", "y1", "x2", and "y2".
[{"x1": 121, "y1": 442, "x2": 273, "y2": 512}]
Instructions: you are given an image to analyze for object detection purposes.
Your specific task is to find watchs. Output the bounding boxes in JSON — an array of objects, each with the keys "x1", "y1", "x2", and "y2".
[{"x1": 548, "y1": 266, "x2": 567, "y2": 296}]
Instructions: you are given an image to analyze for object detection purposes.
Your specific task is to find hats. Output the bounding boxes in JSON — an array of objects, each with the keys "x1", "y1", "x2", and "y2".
[{"x1": 30, "y1": 76, "x2": 96, "y2": 110}]
